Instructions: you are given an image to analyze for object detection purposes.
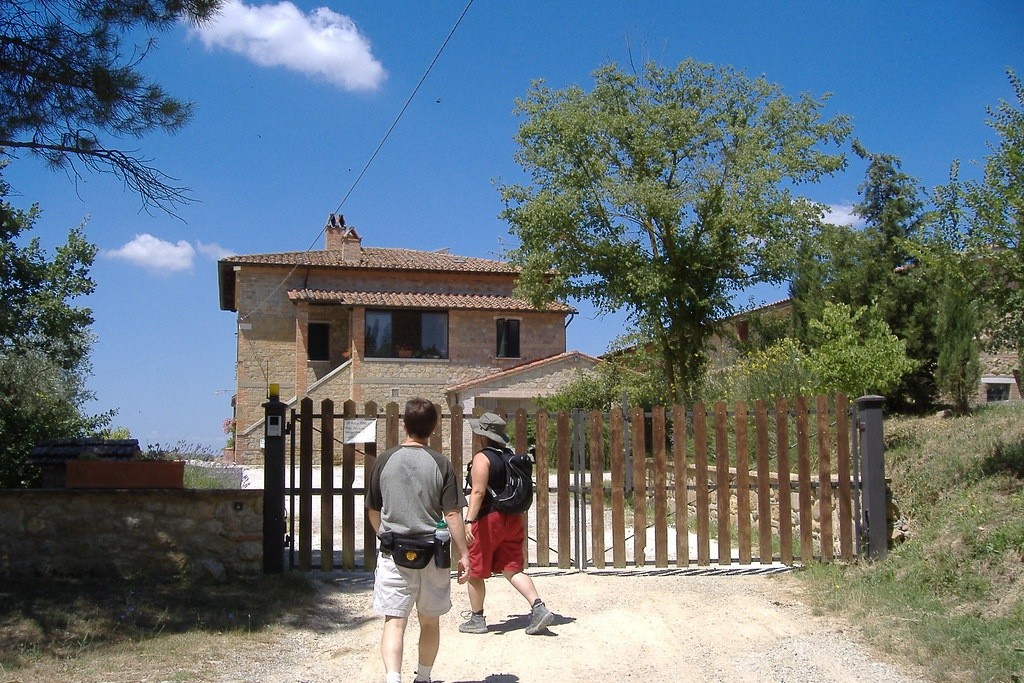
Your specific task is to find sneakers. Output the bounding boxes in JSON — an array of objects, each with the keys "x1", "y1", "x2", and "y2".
[
  {"x1": 525, "y1": 602, "x2": 555, "y2": 634},
  {"x1": 459, "y1": 610, "x2": 488, "y2": 633}
]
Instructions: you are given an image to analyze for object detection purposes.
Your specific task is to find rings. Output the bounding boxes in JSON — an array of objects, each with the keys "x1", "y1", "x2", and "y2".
[{"x1": 468, "y1": 576, "x2": 470, "y2": 578}]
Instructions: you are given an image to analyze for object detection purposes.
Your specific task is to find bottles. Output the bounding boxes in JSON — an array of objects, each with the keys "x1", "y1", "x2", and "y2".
[{"x1": 435, "y1": 520, "x2": 450, "y2": 544}]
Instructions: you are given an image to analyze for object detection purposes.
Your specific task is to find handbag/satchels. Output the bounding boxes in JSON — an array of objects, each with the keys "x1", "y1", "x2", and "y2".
[{"x1": 378, "y1": 532, "x2": 435, "y2": 569}]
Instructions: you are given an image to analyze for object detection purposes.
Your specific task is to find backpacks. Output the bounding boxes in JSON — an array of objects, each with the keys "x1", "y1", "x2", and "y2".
[{"x1": 475, "y1": 446, "x2": 536, "y2": 515}]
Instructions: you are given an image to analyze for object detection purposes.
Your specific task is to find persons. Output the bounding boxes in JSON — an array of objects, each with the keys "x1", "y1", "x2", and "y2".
[
  {"x1": 365, "y1": 398, "x2": 472, "y2": 683},
  {"x1": 459, "y1": 413, "x2": 555, "y2": 635}
]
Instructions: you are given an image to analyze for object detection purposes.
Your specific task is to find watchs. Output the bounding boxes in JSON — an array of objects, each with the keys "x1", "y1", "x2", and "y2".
[{"x1": 465, "y1": 519, "x2": 472, "y2": 524}]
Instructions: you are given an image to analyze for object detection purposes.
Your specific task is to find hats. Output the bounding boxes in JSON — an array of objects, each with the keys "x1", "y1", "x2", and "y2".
[{"x1": 468, "y1": 413, "x2": 510, "y2": 443}]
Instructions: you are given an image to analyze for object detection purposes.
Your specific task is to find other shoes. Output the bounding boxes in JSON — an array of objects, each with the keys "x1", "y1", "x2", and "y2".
[{"x1": 413, "y1": 679, "x2": 431, "y2": 683}]
